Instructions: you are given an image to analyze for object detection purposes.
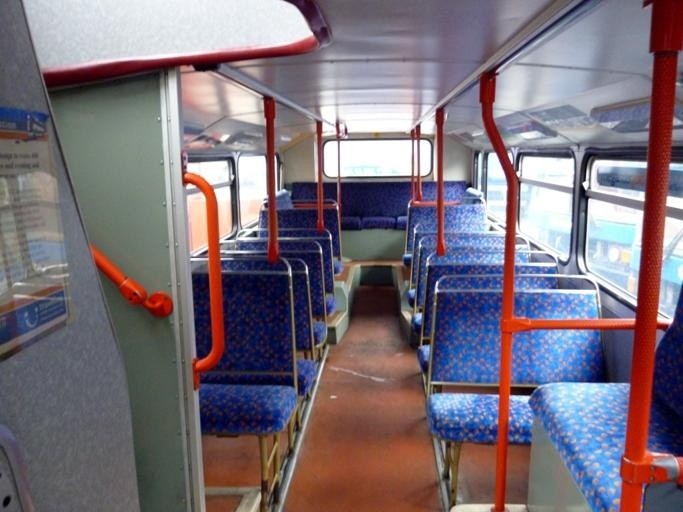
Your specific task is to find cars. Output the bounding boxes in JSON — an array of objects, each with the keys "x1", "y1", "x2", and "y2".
[{"x1": 489, "y1": 168, "x2": 682, "y2": 317}]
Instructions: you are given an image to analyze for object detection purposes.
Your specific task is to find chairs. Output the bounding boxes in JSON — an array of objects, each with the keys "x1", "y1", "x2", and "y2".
[
  {"x1": 363, "y1": 183, "x2": 396, "y2": 230},
  {"x1": 397, "y1": 181, "x2": 682, "y2": 510},
  {"x1": 193, "y1": 180, "x2": 363, "y2": 509}
]
